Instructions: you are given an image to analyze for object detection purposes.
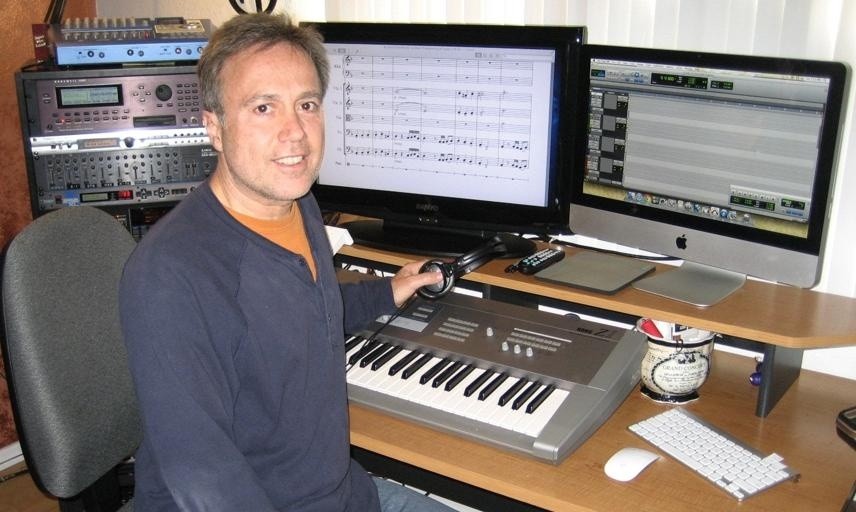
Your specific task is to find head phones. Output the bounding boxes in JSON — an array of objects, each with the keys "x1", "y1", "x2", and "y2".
[{"x1": 416, "y1": 242, "x2": 493, "y2": 301}]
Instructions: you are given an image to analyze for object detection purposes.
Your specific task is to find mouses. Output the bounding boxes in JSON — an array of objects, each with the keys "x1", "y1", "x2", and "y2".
[{"x1": 604, "y1": 447, "x2": 660, "y2": 482}]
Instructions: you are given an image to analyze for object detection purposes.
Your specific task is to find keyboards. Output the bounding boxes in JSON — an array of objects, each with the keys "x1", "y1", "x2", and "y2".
[{"x1": 627, "y1": 405, "x2": 801, "y2": 502}]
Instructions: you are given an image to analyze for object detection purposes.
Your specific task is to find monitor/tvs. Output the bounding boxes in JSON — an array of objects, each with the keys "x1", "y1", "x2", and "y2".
[
  {"x1": 297, "y1": 20, "x2": 588, "y2": 261},
  {"x1": 567, "y1": 43, "x2": 852, "y2": 309}
]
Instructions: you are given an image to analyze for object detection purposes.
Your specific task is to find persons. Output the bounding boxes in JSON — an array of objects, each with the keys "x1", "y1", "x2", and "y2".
[{"x1": 116, "y1": 7, "x2": 459, "y2": 512}]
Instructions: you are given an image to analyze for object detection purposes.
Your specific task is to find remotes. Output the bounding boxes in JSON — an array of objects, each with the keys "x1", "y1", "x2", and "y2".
[{"x1": 517, "y1": 247, "x2": 564, "y2": 275}]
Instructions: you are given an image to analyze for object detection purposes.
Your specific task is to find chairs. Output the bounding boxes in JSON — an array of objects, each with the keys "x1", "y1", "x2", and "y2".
[{"x1": 0, "y1": 205, "x2": 138, "y2": 511}]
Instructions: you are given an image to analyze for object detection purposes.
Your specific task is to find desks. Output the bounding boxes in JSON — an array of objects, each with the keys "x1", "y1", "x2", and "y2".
[{"x1": 338, "y1": 215, "x2": 855, "y2": 511}]
